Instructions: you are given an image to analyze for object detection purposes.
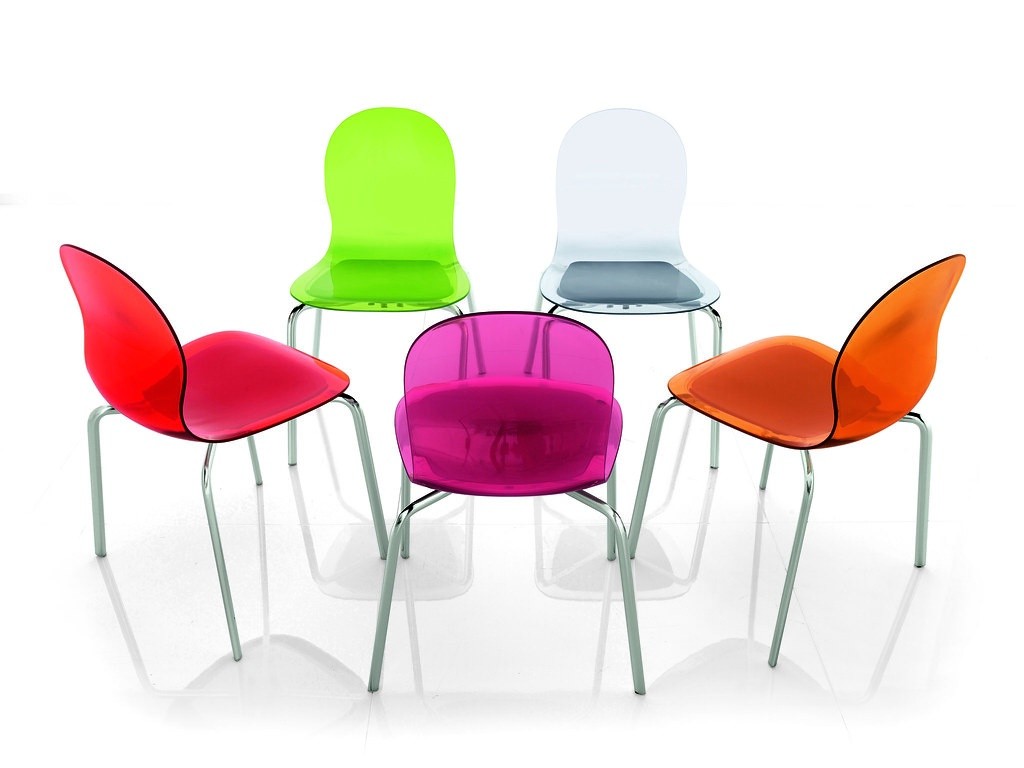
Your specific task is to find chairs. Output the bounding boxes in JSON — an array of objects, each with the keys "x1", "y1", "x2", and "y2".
[
  {"x1": 628, "y1": 252, "x2": 967, "y2": 667},
  {"x1": 522, "y1": 105, "x2": 721, "y2": 467},
  {"x1": 364, "y1": 309, "x2": 645, "y2": 695},
  {"x1": 287, "y1": 108, "x2": 487, "y2": 470},
  {"x1": 59, "y1": 243, "x2": 388, "y2": 662}
]
